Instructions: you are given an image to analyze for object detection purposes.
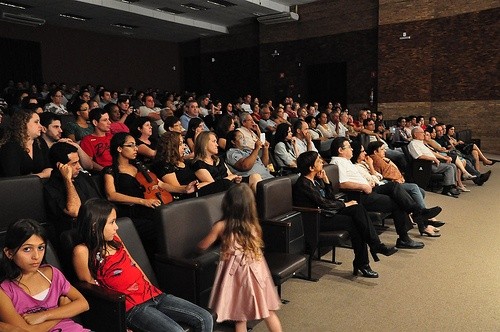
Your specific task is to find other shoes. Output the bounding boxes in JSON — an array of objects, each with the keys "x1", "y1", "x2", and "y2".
[
  {"x1": 463, "y1": 172, "x2": 477, "y2": 181},
  {"x1": 457, "y1": 186, "x2": 470, "y2": 192},
  {"x1": 483, "y1": 160, "x2": 496, "y2": 166},
  {"x1": 471, "y1": 170, "x2": 491, "y2": 186},
  {"x1": 441, "y1": 187, "x2": 461, "y2": 197}
]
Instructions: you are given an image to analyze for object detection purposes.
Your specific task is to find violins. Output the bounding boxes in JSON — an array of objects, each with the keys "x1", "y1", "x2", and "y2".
[{"x1": 133, "y1": 158, "x2": 173, "y2": 205}]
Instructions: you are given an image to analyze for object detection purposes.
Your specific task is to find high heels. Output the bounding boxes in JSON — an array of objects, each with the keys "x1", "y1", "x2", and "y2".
[
  {"x1": 425, "y1": 219, "x2": 446, "y2": 228},
  {"x1": 419, "y1": 224, "x2": 441, "y2": 237},
  {"x1": 370, "y1": 243, "x2": 398, "y2": 262},
  {"x1": 353, "y1": 260, "x2": 378, "y2": 278}
]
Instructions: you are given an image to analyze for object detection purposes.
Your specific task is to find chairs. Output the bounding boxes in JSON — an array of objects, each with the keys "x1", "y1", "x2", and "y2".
[{"x1": 0, "y1": 118, "x2": 482, "y2": 332}]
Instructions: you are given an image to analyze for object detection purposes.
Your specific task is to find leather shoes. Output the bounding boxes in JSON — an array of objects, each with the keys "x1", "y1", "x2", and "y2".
[
  {"x1": 396, "y1": 238, "x2": 424, "y2": 249},
  {"x1": 411, "y1": 205, "x2": 442, "y2": 223}
]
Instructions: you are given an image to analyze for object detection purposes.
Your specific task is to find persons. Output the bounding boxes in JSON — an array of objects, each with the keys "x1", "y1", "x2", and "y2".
[
  {"x1": 185, "y1": 117, "x2": 206, "y2": 152},
  {"x1": 329, "y1": 137, "x2": 442, "y2": 249},
  {"x1": 259, "y1": 107, "x2": 278, "y2": 136},
  {"x1": 42, "y1": 89, "x2": 68, "y2": 115},
  {"x1": 0, "y1": 79, "x2": 500, "y2": 332},
  {"x1": 304, "y1": 115, "x2": 338, "y2": 159},
  {"x1": 180, "y1": 101, "x2": 210, "y2": 131},
  {"x1": 270, "y1": 123, "x2": 301, "y2": 174},
  {"x1": 130, "y1": 116, "x2": 159, "y2": 162},
  {"x1": 62, "y1": 99, "x2": 93, "y2": 143},
  {"x1": 294, "y1": 150, "x2": 399, "y2": 277},
  {"x1": 225, "y1": 130, "x2": 275, "y2": 183},
  {"x1": 101, "y1": 132, "x2": 200, "y2": 216},
  {"x1": 409, "y1": 126, "x2": 460, "y2": 198},
  {"x1": 79, "y1": 107, "x2": 114, "y2": 170}
]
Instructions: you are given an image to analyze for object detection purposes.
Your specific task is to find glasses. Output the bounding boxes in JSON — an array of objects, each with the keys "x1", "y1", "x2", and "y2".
[
  {"x1": 79, "y1": 106, "x2": 88, "y2": 111},
  {"x1": 123, "y1": 144, "x2": 137, "y2": 147},
  {"x1": 54, "y1": 94, "x2": 63, "y2": 98}
]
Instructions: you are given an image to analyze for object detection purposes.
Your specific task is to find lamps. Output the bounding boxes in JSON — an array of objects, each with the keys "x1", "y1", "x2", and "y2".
[{"x1": 256, "y1": 4, "x2": 300, "y2": 26}]
